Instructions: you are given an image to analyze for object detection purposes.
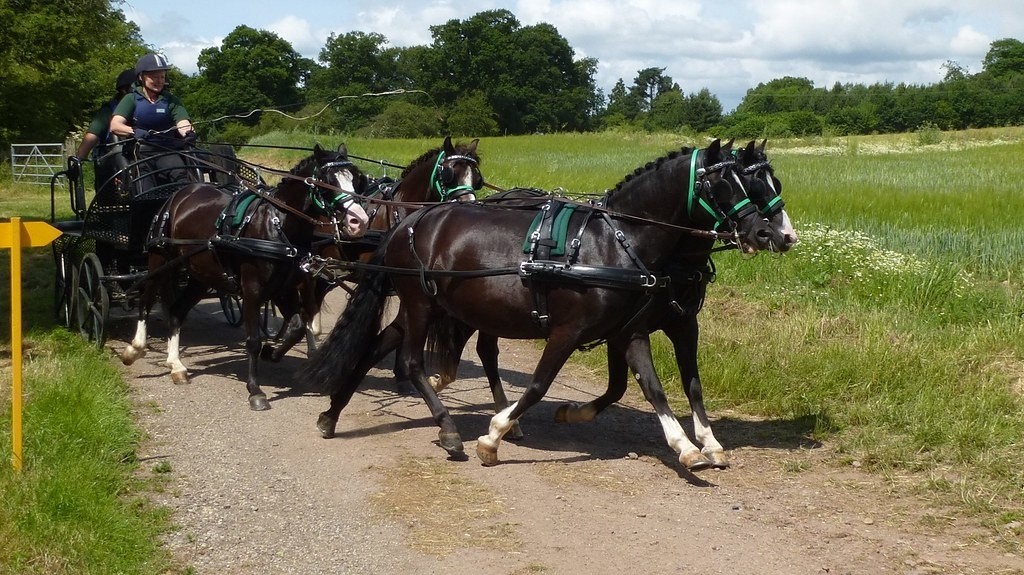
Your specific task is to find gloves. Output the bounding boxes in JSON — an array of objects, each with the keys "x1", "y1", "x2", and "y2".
[
  {"x1": 183, "y1": 131, "x2": 197, "y2": 142},
  {"x1": 67, "y1": 164, "x2": 80, "y2": 181},
  {"x1": 133, "y1": 128, "x2": 151, "y2": 141}
]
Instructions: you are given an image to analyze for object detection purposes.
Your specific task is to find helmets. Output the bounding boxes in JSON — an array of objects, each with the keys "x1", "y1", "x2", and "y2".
[
  {"x1": 116, "y1": 70, "x2": 137, "y2": 88},
  {"x1": 135, "y1": 53, "x2": 171, "y2": 77}
]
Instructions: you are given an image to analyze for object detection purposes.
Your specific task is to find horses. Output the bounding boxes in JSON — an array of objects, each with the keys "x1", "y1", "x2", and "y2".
[
  {"x1": 316, "y1": 137, "x2": 798, "y2": 476},
  {"x1": 120, "y1": 138, "x2": 486, "y2": 413}
]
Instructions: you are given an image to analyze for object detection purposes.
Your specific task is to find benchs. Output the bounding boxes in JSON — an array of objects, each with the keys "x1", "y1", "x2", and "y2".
[{"x1": 103, "y1": 134, "x2": 242, "y2": 206}]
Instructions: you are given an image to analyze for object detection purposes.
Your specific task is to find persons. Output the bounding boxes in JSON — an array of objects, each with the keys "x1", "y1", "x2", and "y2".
[{"x1": 78, "y1": 53, "x2": 197, "y2": 205}]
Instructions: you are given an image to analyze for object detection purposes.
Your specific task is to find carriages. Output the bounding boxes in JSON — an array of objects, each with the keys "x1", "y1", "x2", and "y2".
[{"x1": 50, "y1": 121, "x2": 797, "y2": 473}]
